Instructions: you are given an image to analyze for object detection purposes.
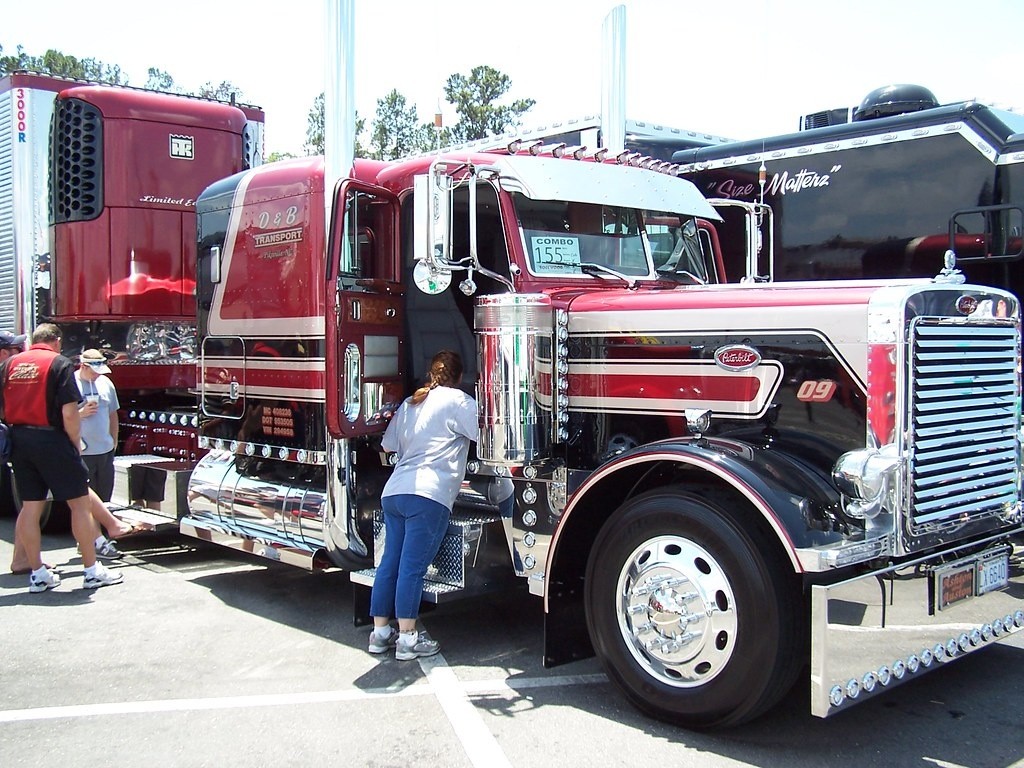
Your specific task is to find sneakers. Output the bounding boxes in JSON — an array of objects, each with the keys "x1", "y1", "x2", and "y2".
[
  {"x1": 396, "y1": 630, "x2": 441, "y2": 660},
  {"x1": 369, "y1": 627, "x2": 399, "y2": 653},
  {"x1": 76, "y1": 538, "x2": 125, "y2": 559},
  {"x1": 83, "y1": 561, "x2": 124, "y2": 588},
  {"x1": 29, "y1": 569, "x2": 61, "y2": 592}
]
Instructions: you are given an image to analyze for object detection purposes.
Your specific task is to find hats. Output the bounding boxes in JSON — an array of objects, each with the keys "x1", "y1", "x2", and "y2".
[
  {"x1": 0, "y1": 331, "x2": 27, "y2": 349},
  {"x1": 78, "y1": 354, "x2": 112, "y2": 375}
]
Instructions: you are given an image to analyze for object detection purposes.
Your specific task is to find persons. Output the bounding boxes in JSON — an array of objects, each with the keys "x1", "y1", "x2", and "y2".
[
  {"x1": 0, "y1": 323, "x2": 149, "y2": 592},
  {"x1": 368, "y1": 349, "x2": 479, "y2": 660}
]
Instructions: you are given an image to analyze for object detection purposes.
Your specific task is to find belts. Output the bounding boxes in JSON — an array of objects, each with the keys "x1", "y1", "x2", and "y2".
[{"x1": 13, "y1": 424, "x2": 64, "y2": 431}]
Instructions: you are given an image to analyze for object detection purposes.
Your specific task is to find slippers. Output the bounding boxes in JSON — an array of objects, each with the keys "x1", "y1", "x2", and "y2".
[
  {"x1": 114, "y1": 522, "x2": 157, "y2": 539},
  {"x1": 13, "y1": 562, "x2": 57, "y2": 575}
]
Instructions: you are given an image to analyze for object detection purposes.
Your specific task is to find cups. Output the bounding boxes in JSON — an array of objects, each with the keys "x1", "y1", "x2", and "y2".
[{"x1": 84, "y1": 393, "x2": 99, "y2": 409}]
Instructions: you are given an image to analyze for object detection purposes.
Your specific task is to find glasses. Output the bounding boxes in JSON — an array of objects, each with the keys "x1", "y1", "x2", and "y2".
[
  {"x1": 1, "y1": 344, "x2": 22, "y2": 353},
  {"x1": 83, "y1": 360, "x2": 108, "y2": 365}
]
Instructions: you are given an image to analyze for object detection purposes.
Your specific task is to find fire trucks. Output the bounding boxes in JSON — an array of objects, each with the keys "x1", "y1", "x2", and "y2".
[{"x1": 0, "y1": 67, "x2": 1024, "y2": 736}]
